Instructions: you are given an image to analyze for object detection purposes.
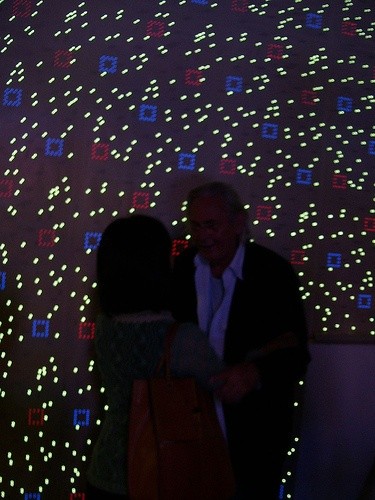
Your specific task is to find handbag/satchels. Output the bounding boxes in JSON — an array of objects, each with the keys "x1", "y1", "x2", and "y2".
[{"x1": 127, "y1": 323, "x2": 235, "y2": 500}]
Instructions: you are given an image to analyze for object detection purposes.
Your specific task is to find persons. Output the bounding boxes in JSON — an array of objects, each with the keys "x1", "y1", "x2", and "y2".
[
  {"x1": 171, "y1": 183, "x2": 305, "y2": 499},
  {"x1": 84, "y1": 215, "x2": 242, "y2": 499}
]
